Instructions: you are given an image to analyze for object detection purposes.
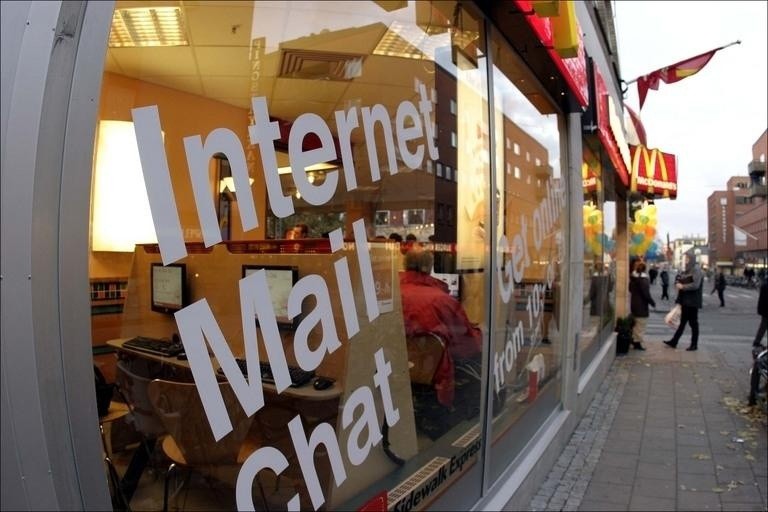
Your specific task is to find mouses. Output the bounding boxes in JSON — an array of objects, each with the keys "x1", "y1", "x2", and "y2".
[
  {"x1": 314, "y1": 378, "x2": 332, "y2": 390},
  {"x1": 177, "y1": 353, "x2": 187, "y2": 359}
]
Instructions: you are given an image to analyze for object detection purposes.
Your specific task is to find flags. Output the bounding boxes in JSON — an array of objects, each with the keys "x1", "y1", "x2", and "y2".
[{"x1": 637, "y1": 46, "x2": 724, "y2": 112}]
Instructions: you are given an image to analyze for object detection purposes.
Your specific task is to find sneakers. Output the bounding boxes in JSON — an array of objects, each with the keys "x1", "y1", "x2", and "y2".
[
  {"x1": 661, "y1": 338, "x2": 677, "y2": 350},
  {"x1": 683, "y1": 344, "x2": 698, "y2": 352}
]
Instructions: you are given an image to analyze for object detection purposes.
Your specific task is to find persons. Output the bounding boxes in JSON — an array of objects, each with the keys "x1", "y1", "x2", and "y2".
[
  {"x1": 399, "y1": 248, "x2": 483, "y2": 421},
  {"x1": 629, "y1": 249, "x2": 727, "y2": 350},
  {"x1": 292, "y1": 223, "x2": 308, "y2": 245},
  {"x1": 588, "y1": 261, "x2": 613, "y2": 314},
  {"x1": 754, "y1": 268, "x2": 767, "y2": 347}
]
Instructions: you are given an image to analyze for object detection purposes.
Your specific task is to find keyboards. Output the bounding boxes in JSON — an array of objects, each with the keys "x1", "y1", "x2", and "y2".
[
  {"x1": 122, "y1": 336, "x2": 184, "y2": 357},
  {"x1": 217, "y1": 358, "x2": 315, "y2": 388}
]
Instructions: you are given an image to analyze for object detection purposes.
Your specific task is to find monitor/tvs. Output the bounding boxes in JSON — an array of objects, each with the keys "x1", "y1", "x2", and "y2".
[
  {"x1": 150, "y1": 262, "x2": 186, "y2": 316},
  {"x1": 403, "y1": 209, "x2": 425, "y2": 225},
  {"x1": 429, "y1": 264, "x2": 460, "y2": 300},
  {"x1": 242, "y1": 264, "x2": 297, "y2": 330},
  {"x1": 375, "y1": 210, "x2": 390, "y2": 226}
]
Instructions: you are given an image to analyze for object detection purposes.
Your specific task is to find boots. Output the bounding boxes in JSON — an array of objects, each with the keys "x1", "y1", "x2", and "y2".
[{"x1": 632, "y1": 340, "x2": 646, "y2": 351}]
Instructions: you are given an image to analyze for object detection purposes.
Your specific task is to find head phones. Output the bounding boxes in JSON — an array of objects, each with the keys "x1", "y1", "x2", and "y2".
[{"x1": 168, "y1": 333, "x2": 184, "y2": 353}]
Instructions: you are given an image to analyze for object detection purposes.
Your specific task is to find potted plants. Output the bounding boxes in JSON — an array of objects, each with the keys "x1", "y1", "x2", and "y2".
[{"x1": 615, "y1": 315, "x2": 637, "y2": 355}]
